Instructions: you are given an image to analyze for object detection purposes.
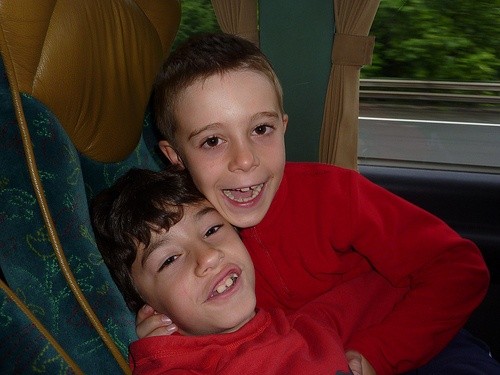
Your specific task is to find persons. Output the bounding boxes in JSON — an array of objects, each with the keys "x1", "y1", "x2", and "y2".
[
  {"x1": 90, "y1": 166, "x2": 359, "y2": 375},
  {"x1": 134, "y1": 31, "x2": 500, "y2": 374}
]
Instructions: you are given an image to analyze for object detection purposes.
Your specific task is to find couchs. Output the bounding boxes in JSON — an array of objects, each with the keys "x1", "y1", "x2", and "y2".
[{"x1": 0, "y1": 0, "x2": 181, "y2": 375}]
